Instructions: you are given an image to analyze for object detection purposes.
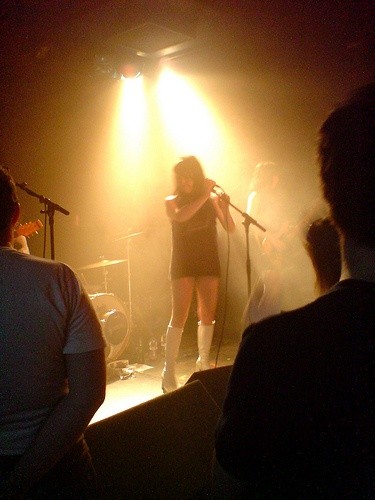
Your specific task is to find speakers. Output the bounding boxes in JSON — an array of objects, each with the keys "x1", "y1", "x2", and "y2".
[
  {"x1": 183, "y1": 365, "x2": 232, "y2": 405},
  {"x1": 82, "y1": 381, "x2": 223, "y2": 499}
]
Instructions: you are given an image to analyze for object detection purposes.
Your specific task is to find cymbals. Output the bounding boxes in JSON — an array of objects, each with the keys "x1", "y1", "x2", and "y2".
[
  {"x1": 119, "y1": 232, "x2": 142, "y2": 240},
  {"x1": 78, "y1": 260, "x2": 127, "y2": 270}
]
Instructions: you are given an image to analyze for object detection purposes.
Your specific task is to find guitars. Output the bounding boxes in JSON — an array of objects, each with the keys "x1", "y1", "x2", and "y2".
[
  {"x1": 261, "y1": 200, "x2": 324, "y2": 271},
  {"x1": 14, "y1": 219, "x2": 43, "y2": 239}
]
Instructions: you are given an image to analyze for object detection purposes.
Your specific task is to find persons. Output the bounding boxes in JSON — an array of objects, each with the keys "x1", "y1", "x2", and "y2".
[
  {"x1": 306, "y1": 215, "x2": 340, "y2": 299},
  {"x1": 241, "y1": 162, "x2": 288, "y2": 330},
  {"x1": 161, "y1": 157, "x2": 234, "y2": 394},
  {"x1": 209, "y1": 85, "x2": 375, "y2": 500},
  {"x1": 0, "y1": 168, "x2": 105, "y2": 500}
]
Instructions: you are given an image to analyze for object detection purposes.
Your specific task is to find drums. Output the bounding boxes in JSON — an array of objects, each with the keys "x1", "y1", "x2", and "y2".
[{"x1": 88, "y1": 292, "x2": 130, "y2": 364}]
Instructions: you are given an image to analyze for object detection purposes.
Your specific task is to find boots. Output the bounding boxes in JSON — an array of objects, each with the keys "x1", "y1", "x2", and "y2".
[
  {"x1": 162, "y1": 325, "x2": 183, "y2": 394},
  {"x1": 196, "y1": 325, "x2": 214, "y2": 373}
]
